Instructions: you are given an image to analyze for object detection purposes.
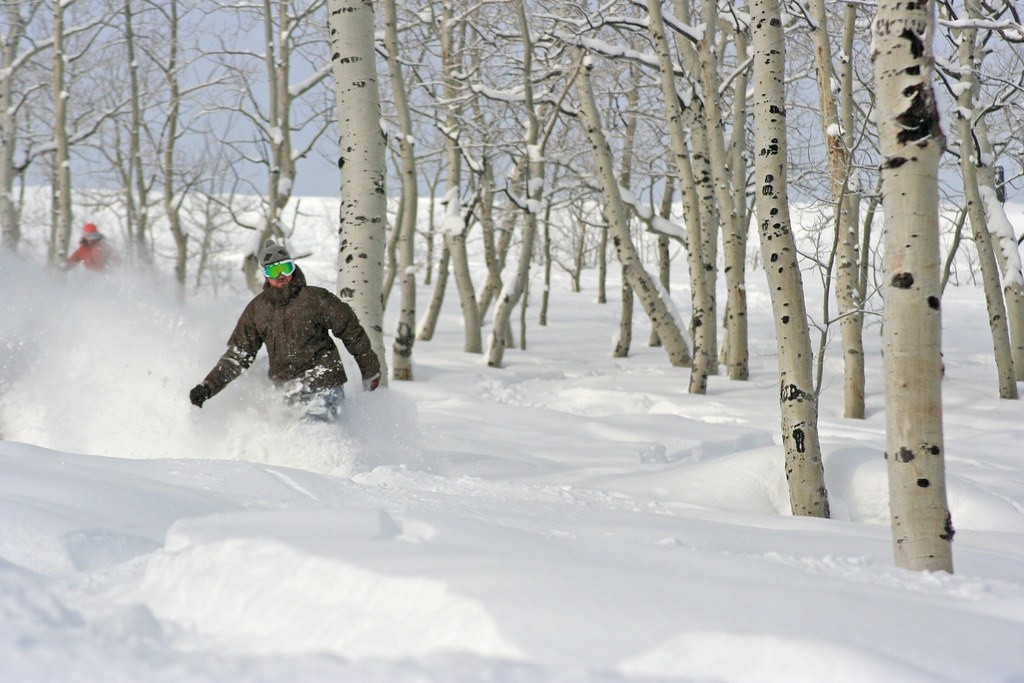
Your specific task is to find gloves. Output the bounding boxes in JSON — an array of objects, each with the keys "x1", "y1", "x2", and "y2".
[
  {"x1": 362, "y1": 373, "x2": 384, "y2": 391},
  {"x1": 190, "y1": 384, "x2": 212, "y2": 408}
]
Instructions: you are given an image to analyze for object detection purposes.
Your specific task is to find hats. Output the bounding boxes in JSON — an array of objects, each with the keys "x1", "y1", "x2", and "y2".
[
  {"x1": 257, "y1": 239, "x2": 291, "y2": 262},
  {"x1": 81, "y1": 224, "x2": 99, "y2": 239}
]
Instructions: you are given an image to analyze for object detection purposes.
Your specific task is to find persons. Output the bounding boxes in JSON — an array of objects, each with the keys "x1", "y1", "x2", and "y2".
[
  {"x1": 69, "y1": 223, "x2": 119, "y2": 272},
  {"x1": 190, "y1": 239, "x2": 381, "y2": 410}
]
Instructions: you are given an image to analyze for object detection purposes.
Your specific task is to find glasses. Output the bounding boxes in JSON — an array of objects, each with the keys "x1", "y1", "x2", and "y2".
[{"x1": 261, "y1": 257, "x2": 296, "y2": 279}]
